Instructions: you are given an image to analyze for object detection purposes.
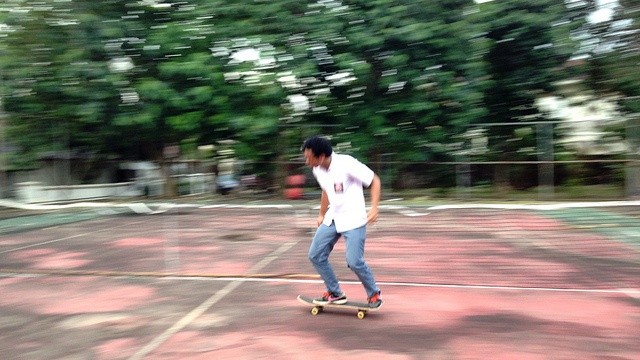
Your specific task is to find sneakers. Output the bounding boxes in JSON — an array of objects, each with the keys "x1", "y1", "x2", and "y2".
[
  {"x1": 367, "y1": 290, "x2": 383, "y2": 311},
  {"x1": 313, "y1": 291, "x2": 348, "y2": 305}
]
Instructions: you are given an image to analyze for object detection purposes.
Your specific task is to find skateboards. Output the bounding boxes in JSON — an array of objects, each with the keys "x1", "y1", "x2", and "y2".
[{"x1": 297, "y1": 293, "x2": 382, "y2": 319}]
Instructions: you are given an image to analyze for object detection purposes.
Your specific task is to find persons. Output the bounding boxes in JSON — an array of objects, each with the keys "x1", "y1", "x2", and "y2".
[{"x1": 300, "y1": 136, "x2": 383, "y2": 308}]
